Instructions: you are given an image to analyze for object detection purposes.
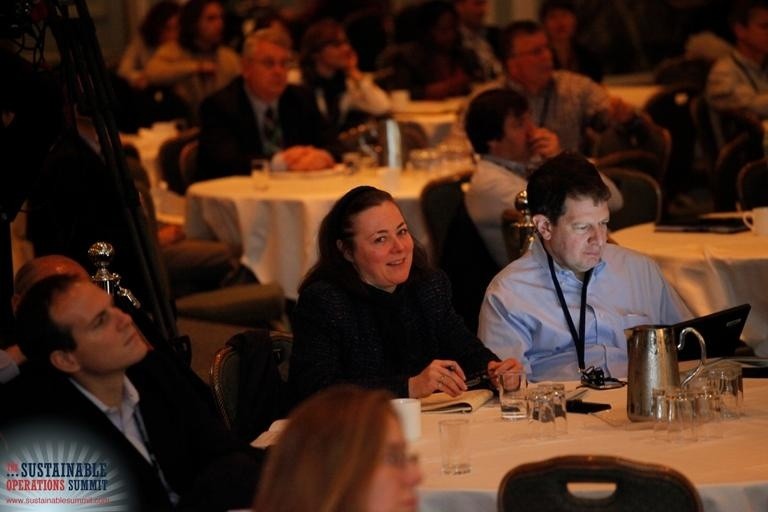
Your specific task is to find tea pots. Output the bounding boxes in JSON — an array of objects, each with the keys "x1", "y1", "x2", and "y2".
[
  {"x1": 359, "y1": 114, "x2": 407, "y2": 169},
  {"x1": 621, "y1": 323, "x2": 706, "y2": 424}
]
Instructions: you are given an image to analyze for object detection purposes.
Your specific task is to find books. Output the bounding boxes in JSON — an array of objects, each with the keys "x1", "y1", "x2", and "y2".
[
  {"x1": 420, "y1": 390, "x2": 493, "y2": 416},
  {"x1": 651, "y1": 216, "x2": 754, "y2": 235}
]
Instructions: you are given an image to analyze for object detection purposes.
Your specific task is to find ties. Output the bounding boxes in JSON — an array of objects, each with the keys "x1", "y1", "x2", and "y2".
[{"x1": 257, "y1": 105, "x2": 280, "y2": 159}]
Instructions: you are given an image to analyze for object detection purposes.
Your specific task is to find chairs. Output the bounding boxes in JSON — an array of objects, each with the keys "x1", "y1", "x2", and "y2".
[
  {"x1": 642, "y1": 82, "x2": 703, "y2": 170},
  {"x1": 179, "y1": 136, "x2": 200, "y2": 182},
  {"x1": 599, "y1": 149, "x2": 658, "y2": 168},
  {"x1": 209, "y1": 330, "x2": 293, "y2": 490},
  {"x1": 418, "y1": 169, "x2": 502, "y2": 335},
  {"x1": 709, "y1": 104, "x2": 764, "y2": 212},
  {"x1": 735, "y1": 157, "x2": 767, "y2": 210},
  {"x1": 603, "y1": 166, "x2": 662, "y2": 232},
  {"x1": 126, "y1": 144, "x2": 240, "y2": 281},
  {"x1": 496, "y1": 454, "x2": 704, "y2": 511}
]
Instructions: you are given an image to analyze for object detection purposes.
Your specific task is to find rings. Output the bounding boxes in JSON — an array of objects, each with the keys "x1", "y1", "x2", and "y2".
[{"x1": 438, "y1": 376, "x2": 448, "y2": 384}]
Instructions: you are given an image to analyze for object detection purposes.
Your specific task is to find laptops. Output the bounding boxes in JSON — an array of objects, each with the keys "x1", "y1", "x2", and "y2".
[{"x1": 662, "y1": 301, "x2": 752, "y2": 361}]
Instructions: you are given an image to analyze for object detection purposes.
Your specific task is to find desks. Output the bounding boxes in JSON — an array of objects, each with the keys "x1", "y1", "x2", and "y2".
[
  {"x1": 183, "y1": 165, "x2": 470, "y2": 301},
  {"x1": 393, "y1": 111, "x2": 457, "y2": 149},
  {"x1": 608, "y1": 210, "x2": 768, "y2": 358},
  {"x1": 402, "y1": 375, "x2": 768, "y2": 512}
]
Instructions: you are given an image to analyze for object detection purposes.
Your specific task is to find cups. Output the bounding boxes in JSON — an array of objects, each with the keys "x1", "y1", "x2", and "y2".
[
  {"x1": 440, "y1": 420, "x2": 474, "y2": 476},
  {"x1": 527, "y1": 386, "x2": 557, "y2": 444},
  {"x1": 391, "y1": 397, "x2": 422, "y2": 449},
  {"x1": 250, "y1": 160, "x2": 270, "y2": 192},
  {"x1": 410, "y1": 141, "x2": 463, "y2": 171},
  {"x1": 499, "y1": 372, "x2": 528, "y2": 421},
  {"x1": 653, "y1": 387, "x2": 722, "y2": 444},
  {"x1": 706, "y1": 358, "x2": 747, "y2": 420},
  {"x1": 534, "y1": 382, "x2": 569, "y2": 438}
]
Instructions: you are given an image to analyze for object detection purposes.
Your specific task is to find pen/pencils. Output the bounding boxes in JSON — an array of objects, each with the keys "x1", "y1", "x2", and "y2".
[{"x1": 448, "y1": 366, "x2": 454, "y2": 371}]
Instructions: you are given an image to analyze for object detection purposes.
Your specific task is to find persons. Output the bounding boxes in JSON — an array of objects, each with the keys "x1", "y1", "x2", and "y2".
[
  {"x1": 478, "y1": 152, "x2": 694, "y2": 381},
  {"x1": 254, "y1": 385, "x2": 420, "y2": 512},
  {"x1": 1, "y1": 253, "x2": 96, "y2": 386},
  {"x1": 115, "y1": 0, "x2": 767, "y2": 295},
  {"x1": 291, "y1": 184, "x2": 525, "y2": 398},
  {"x1": 0, "y1": 274, "x2": 232, "y2": 512}
]
